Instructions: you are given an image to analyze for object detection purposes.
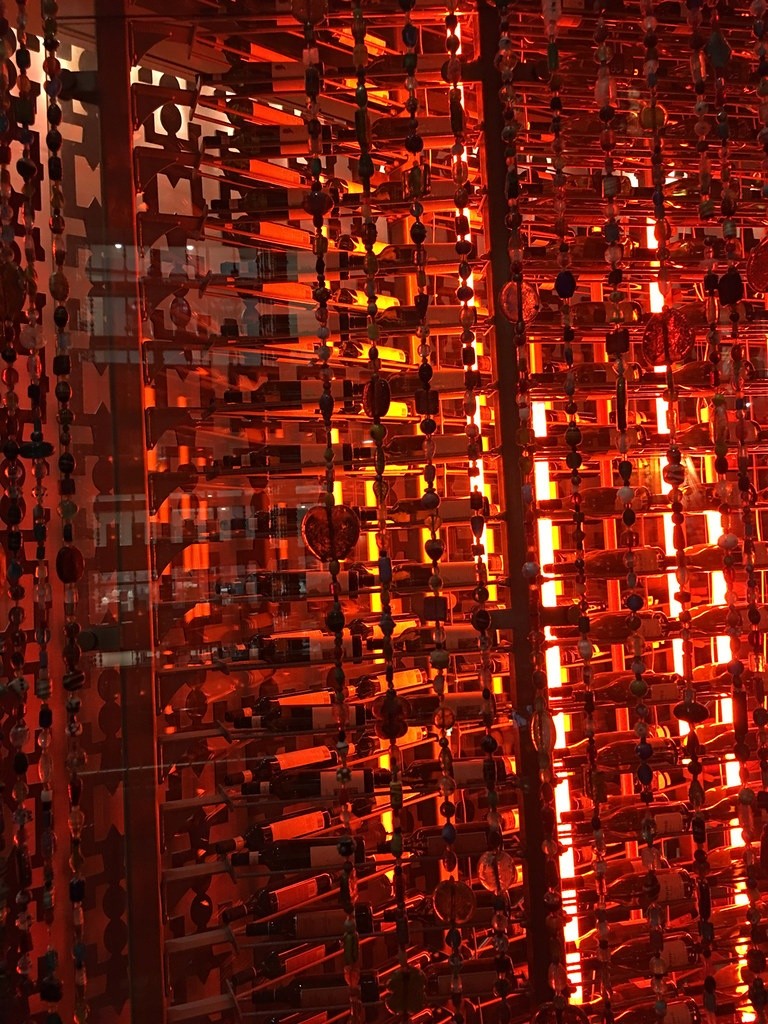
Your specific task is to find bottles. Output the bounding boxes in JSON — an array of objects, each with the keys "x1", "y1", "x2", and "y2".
[{"x1": 196, "y1": 0, "x2": 768, "y2": 1024}]
